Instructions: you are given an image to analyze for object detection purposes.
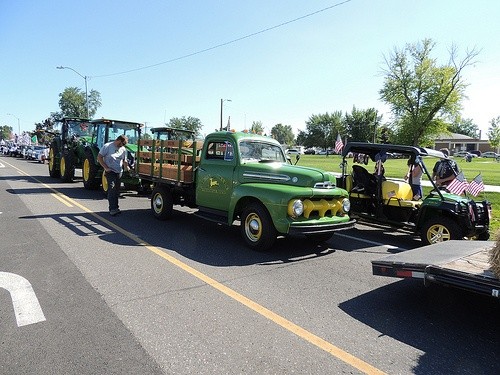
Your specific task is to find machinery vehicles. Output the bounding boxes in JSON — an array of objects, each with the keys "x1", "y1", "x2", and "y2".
[{"x1": 46, "y1": 118, "x2": 148, "y2": 192}]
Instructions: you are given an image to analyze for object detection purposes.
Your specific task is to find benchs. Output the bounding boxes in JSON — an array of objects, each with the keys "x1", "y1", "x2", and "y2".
[
  {"x1": 381, "y1": 180, "x2": 423, "y2": 207},
  {"x1": 346, "y1": 175, "x2": 373, "y2": 199}
]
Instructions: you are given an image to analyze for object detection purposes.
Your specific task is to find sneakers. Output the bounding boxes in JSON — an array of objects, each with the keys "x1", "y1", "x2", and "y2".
[{"x1": 110, "y1": 209, "x2": 121, "y2": 216}]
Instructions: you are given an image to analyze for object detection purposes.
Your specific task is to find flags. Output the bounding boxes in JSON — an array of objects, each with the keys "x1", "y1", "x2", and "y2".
[
  {"x1": 334, "y1": 131, "x2": 344, "y2": 153},
  {"x1": 447, "y1": 168, "x2": 489, "y2": 200}
]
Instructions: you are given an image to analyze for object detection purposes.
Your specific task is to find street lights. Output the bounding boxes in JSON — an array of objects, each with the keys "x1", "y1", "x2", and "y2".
[
  {"x1": 220, "y1": 98, "x2": 232, "y2": 132},
  {"x1": 56, "y1": 66, "x2": 92, "y2": 120}
]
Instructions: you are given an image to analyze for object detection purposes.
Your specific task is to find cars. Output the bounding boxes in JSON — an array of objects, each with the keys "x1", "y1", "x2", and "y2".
[
  {"x1": 1, "y1": 140, "x2": 49, "y2": 163},
  {"x1": 304, "y1": 149, "x2": 316, "y2": 155},
  {"x1": 483, "y1": 152, "x2": 500, "y2": 159},
  {"x1": 318, "y1": 149, "x2": 335, "y2": 154},
  {"x1": 453, "y1": 150, "x2": 481, "y2": 158},
  {"x1": 286, "y1": 150, "x2": 301, "y2": 155}
]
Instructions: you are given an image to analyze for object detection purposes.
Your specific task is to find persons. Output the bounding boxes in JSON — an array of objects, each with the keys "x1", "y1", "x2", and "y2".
[
  {"x1": 407, "y1": 147, "x2": 425, "y2": 199},
  {"x1": 432, "y1": 147, "x2": 459, "y2": 190},
  {"x1": 97, "y1": 134, "x2": 132, "y2": 214}
]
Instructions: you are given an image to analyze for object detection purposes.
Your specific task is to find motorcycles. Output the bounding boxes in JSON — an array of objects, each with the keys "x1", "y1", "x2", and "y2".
[{"x1": 341, "y1": 140, "x2": 493, "y2": 249}]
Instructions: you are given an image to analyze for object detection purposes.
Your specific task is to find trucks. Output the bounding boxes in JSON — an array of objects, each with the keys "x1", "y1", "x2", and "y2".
[{"x1": 132, "y1": 126, "x2": 357, "y2": 252}]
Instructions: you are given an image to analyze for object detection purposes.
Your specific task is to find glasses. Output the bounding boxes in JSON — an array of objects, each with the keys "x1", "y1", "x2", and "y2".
[{"x1": 122, "y1": 142, "x2": 125, "y2": 146}]
